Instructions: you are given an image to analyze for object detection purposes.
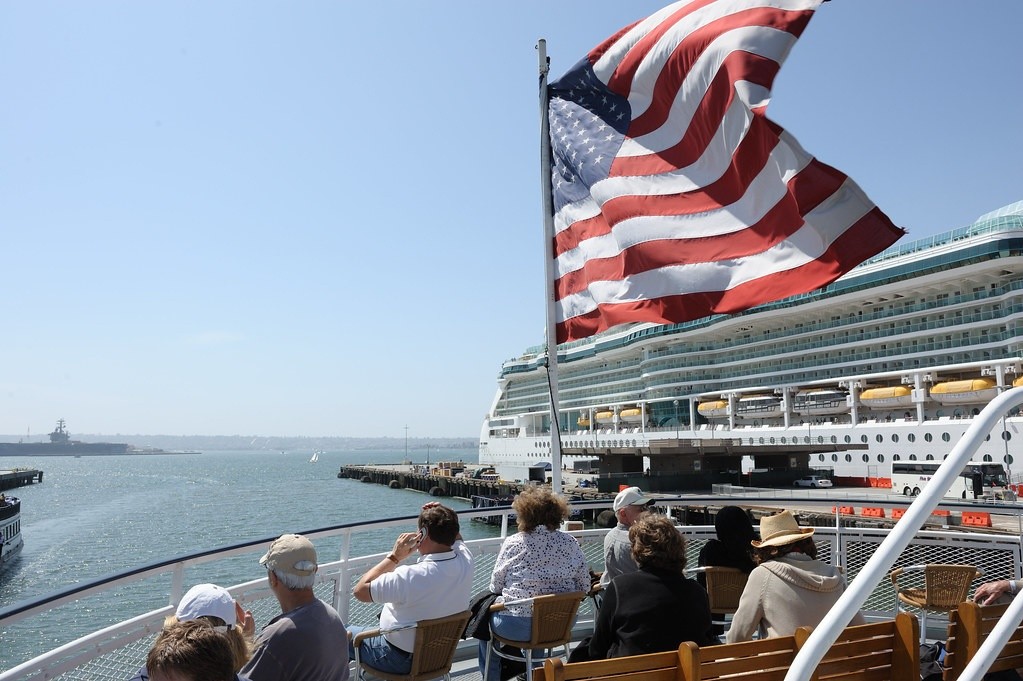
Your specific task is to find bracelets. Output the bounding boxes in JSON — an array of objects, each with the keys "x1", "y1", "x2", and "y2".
[
  {"x1": 387, "y1": 553, "x2": 391, "y2": 565},
  {"x1": 1009, "y1": 580, "x2": 1017, "y2": 595}
]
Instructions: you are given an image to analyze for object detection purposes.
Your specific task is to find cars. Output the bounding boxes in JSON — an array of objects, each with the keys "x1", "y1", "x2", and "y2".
[{"x1": 792, "y1": 475, "x2": 834, "y2": 489}]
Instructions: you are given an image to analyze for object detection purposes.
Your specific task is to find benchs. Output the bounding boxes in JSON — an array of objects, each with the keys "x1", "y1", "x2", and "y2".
[
  {"x1": 533, "y1": 611, "x2": 922, "y2": 681},
  {"x1": 943, "y1": 602, "x2": 1023, "y2": 681}
]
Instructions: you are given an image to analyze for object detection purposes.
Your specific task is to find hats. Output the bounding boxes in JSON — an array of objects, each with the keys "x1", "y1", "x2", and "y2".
[
  {"x1": 613, "y1": 487, "x2": 655, "y2": 512},
  {"x1": 751, "y1": 510, "x2": 815, "y2": 548},
  {"x1": 176, "y1": 583, "x2": 237, "y2": 631},
  {"x1": 259, "y1": 534, "x2": 317, "y2": 577}
]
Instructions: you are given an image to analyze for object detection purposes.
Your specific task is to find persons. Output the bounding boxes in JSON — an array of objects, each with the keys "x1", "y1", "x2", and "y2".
[
  {"x1": 697, "y1": 505, "x2": 762, "y2": 634},
  {"x1": 973, "y1": 577, "x2": 1023, "y2": 606},
  {"x1": 726, "y1": 510, "x2": 872, "y2": 672},
  {"x1": 127, "y1": 583, "x2": 256, "y2": 681},
  {"x1": 646, "y1": 466, "x2": 652, "y2": 476},
  {"x1": 346, "y1": 501, "x2": 474, "y2": 674},
  {"x1": 479, "y1": 481, "x2": 591, "y2": 681},
  {"x1": 566, "y1": 486, "x2": 715, "y2": 664},
  {"x1": 234, "y1": 533, "x2": 350, "y2": 681}
]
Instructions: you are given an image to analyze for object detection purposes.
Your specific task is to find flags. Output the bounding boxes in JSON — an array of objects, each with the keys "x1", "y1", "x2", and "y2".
[{"x1": 546, "y1": 0, "x2": 911, "y2": 347}]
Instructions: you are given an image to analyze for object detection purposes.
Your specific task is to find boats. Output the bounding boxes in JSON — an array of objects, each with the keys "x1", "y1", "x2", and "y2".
[
  {"x1": 619, "y1": 409, "x2": 649, "y2": 423},
  {"x1": 594, "y1": 412, "x2": 615, "y2": 424},
  {"x1": 0, "y1": 493, "x2": 25, "y2": 566},
  {"x1": 697, "y1": 400, "x2": 731, "y2": 419},
  {"x1": 858, "y1": 384, "x2": 916, "y2": 410},
  {"x1": 1012, "y1": 374, "x2": 1023, "y2": 388},
  {"x1": 576, "y1": 415, "x2": 594, "y2": 426},
  {"x1": 928, "y1": 378, "x2": 999, "y2": 404},
  {"x1": 735, "y1": 395, "x2": 785, "y2": 419},
  {"x1": 791, "y1": 387, "x2": 850, "y2": 415}
]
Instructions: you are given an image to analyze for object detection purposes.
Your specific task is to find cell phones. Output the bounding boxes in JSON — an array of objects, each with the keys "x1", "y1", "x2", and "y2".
[{"x1": 409, "y1": 527, "x2": 426, "y2": 548}]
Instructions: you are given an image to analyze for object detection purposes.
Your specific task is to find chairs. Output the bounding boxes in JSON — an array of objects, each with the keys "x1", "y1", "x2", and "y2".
[{"x1": 348, "y1": 565, "x2": 1023, "y2": 681}]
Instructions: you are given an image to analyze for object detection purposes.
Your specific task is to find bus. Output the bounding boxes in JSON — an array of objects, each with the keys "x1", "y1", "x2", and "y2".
[{"x1": 891, "y1": 460, "x2": 1010, "y2": 498}]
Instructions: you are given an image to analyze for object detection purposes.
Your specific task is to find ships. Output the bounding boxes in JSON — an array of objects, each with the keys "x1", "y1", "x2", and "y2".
[{"x1": 477, "y1": 198, "x2": 1023, "y2": 494}]
